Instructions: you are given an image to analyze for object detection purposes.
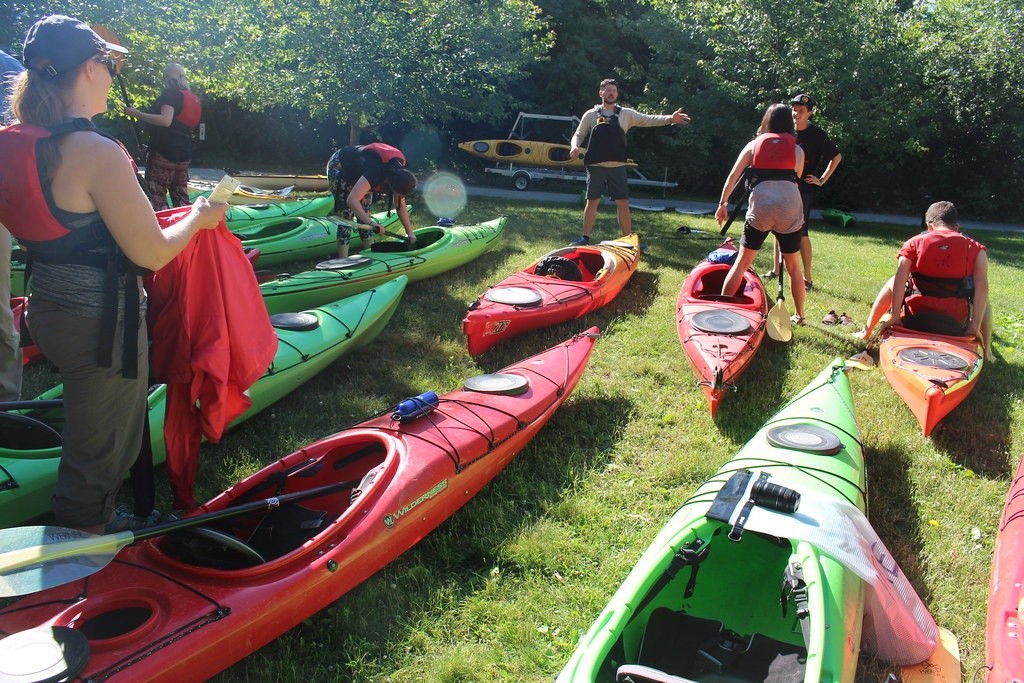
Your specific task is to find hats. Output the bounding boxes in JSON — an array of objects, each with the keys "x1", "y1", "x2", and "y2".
[
  {"x1": 22, "y1": 15, "x2": 129, "y2": 77},
  {"x1": 163, "y1": 63, "x2": 186, "y2": 80},
  {"x1": 788, "y1": 94, "x2": 813, "y2": 110}
]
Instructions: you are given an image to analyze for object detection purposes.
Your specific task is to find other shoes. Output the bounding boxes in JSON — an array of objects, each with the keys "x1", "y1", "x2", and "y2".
[
  {"x1": 839, "y1": 313, "x2": 856, "y2": 328},
  {"x1": 804, "y1": 280, "x2": 814, "y2": 291},
  {"x1": 790, "y1": 314, "x2": 806, "y2": 326},
  {"x1": 569, "y1": 237, "x2": 590, "y2": 247},
  {"x1": 761, "y1": 271, "x2": 779, "y2": 282},
  {"x1": 822, "y1": 311, "x2": 838, "y2": 326}
]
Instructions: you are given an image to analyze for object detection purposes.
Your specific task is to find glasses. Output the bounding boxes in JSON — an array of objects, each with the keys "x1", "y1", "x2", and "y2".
[{"x1": 93, "y1": 57, "x2": 117, "y2": 77}]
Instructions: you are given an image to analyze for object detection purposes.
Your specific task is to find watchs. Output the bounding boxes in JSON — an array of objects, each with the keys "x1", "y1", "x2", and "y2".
[{"x1": 719, "y1": 201, "x2": 728, "y2": 207}]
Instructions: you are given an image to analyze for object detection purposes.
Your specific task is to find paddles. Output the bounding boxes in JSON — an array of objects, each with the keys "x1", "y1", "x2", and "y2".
[
  {"x1": 843, "y1": 312, "x2": 893, "y2": 372},
  {"x1": 0, "y1": 477, "x2": 364, "y2": 599},
  {"x1": 0, "y1": 388, "x2": 71, "y2": 427},
  {"x1": 326, "y1": 214, "x2": 410, "y2": 242},
  {"x1": 765, "y1": 249, "x2": 793, "y2": 342},
  {"x1": 90, "y1": 22, "x2": 146, "y2": 163}
]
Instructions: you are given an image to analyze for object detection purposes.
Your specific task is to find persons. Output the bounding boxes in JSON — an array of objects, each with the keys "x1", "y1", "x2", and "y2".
[
  {"x1": 849, "y1": 200, "x2": 1001, "y2": 365},
  {"x1": 326, "y1": 143, "x2": 418, "y2": 260},
  {"x1": 24, "y1": 13, "x2": 230, "y2": 536},
  {"x1": 569, "y1": 78, "x2": 692, "y2": 247},
  {"x1": 123, "y1": 62, "x2": 196, "y2": 212},
  {"x1": 760, "y1": 94, "x2": 842, "y2": 292},
  {"x1": 0, "y1": 49, "x2": 29, "y2": 402},
  {"x1": 714, "y1": 104, "x2": 805, "y2": 327}
]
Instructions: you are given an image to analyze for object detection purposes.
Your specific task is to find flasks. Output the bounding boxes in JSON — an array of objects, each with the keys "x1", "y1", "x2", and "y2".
[
  {"x1": 392, "y1": 393, "x2": 439, "y2": 423},
  {"x1": 438, "y1": 217, "x2": 455, "y2": 226}
]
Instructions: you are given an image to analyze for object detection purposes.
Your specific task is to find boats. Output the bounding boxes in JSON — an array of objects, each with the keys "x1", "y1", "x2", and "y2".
[
  {"x1": 675, "y1": 232, "x2": 771, "y2": 417},
  {"x1": 0, "y1": 322, "x2": 605, "y2": 683},
  {"x1": 877, "y1": 309, "x2": 987, "y2": 438},
  {"x1": 8, "y1": 170, "x2": 511, "y2": 320},
  {"x1": 460, "y1": 231, "x2": 645, "y2": 357},
  {"x1": 456, "y1": 138, "x2": 640, "y2": 170},
  {"x1": 548, "y1": 354, "x2": 940, "y2": 683},
  {"x1": 968, "y1": 451, "x2": 1024, "y2": 683},
  {"x1": 0, "y1": 272, "x2": 413, "y2": 534}
]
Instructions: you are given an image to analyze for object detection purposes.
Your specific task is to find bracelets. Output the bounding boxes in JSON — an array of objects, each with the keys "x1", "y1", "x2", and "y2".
[
  {"x1": 670, "y1": 117, "x2": 671, "y2": 123},
  {"x1": 972, "y1": 327, "x2": 984, "y2": 335}
]
